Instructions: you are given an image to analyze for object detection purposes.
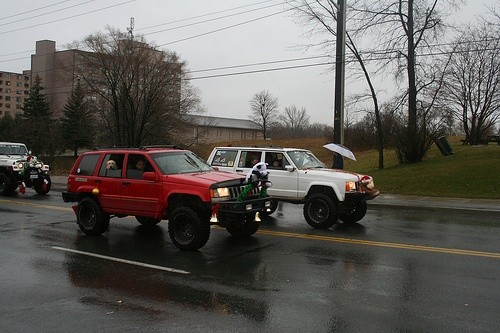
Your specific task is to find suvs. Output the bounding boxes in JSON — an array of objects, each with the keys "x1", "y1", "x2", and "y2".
[
  {"x1": 207, "y1": 145, "x2": 379, "y2": 229},
  {"x1": 62, "y1": 145, "x2": 279, "y2": 252},
  {"x1": 0, "y1": 142, "x2": 52, "y2": 195}
]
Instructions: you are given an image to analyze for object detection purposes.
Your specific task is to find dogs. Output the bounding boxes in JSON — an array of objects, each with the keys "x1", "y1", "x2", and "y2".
[{"x1": 107, "y1": 160, "x2": 117, "y2": 170}]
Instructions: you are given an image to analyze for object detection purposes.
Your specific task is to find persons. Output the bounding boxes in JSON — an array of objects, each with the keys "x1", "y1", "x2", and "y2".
[
  {"x1": 332, "y1": 152, "x2": 343, "y2": 169},
  {"x1": 135, "y1": 158, "x2": 145, "y2": 171},
  {"x1": 246, "y1": 156, "x2": 259, "y2": 168}
]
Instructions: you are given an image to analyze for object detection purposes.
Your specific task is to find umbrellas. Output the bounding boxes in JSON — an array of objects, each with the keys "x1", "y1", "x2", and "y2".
[{"x1": 322, "y1": 143, "x2": 356, "y2": 161}]
